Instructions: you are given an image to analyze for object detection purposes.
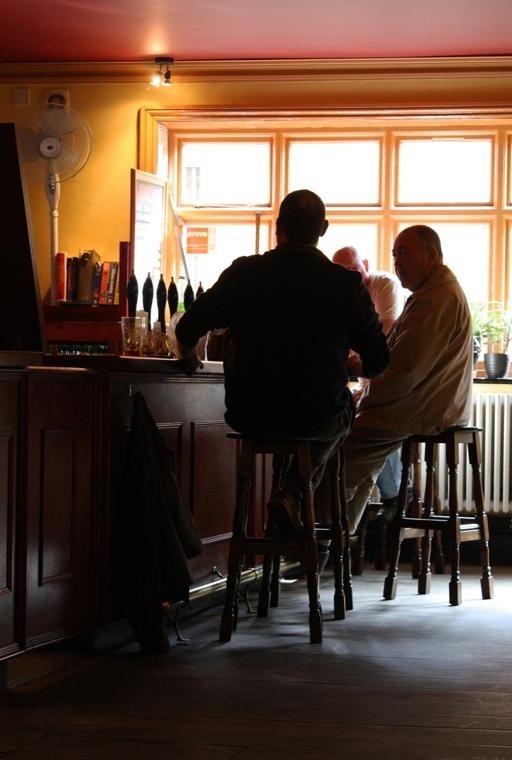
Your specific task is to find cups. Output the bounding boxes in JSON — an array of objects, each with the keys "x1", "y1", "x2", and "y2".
[{"x1": 121, "y1": 317, "x2": 145, "y2": 357}]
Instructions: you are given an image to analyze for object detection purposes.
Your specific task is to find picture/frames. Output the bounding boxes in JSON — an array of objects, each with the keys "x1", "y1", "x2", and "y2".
[{"x1": 131, "y1": 169, "x2": 168, "y2": 308}]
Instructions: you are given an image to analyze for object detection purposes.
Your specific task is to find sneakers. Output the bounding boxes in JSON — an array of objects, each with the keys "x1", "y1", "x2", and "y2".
[{"x1": 266, "y1": 492, "x2": 330, "y2": 574}]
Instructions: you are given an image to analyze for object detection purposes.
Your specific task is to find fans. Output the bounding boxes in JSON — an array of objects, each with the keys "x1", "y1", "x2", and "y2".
[{"x1": 15, "y1": 105, "x2": 94, "y2": 305}]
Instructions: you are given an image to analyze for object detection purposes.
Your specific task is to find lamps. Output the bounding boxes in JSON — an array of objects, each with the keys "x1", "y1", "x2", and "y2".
[{"x1": 150, "y1": 56, "x2": 173, "y2": 87}]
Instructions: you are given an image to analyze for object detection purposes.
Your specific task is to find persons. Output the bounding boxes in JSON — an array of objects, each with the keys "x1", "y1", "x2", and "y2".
[
  {"x1": 283, "y1": 222, "x2": 476, "y2": 580},
  {"x1": 330, "y1": 247, "x2": 408, "y2": 523},
  {"x1": 174, "y1": 192, "x2": 390, "y2": 557}
]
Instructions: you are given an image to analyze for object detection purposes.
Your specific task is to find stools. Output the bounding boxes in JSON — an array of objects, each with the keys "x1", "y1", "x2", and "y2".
[
  {"x1": 220, "y1": 427, "x2": 355, "y2": 644},
  {"x1": 382, "y1": 428, "x2": 493, "y2": 604}
]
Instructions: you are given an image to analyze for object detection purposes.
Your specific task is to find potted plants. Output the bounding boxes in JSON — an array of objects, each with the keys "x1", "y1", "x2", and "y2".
[
  {"x1": 481, "y1": 301, "x2": 512, "y2": 380},
  {"x1": 470, "y1": 309, "x2": 487, "y2": 378}
]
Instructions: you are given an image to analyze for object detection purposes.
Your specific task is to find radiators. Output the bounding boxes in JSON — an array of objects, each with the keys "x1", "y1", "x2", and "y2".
[{"x1": 417, "y1": 395, "x2": 512, "y2": 518}]
